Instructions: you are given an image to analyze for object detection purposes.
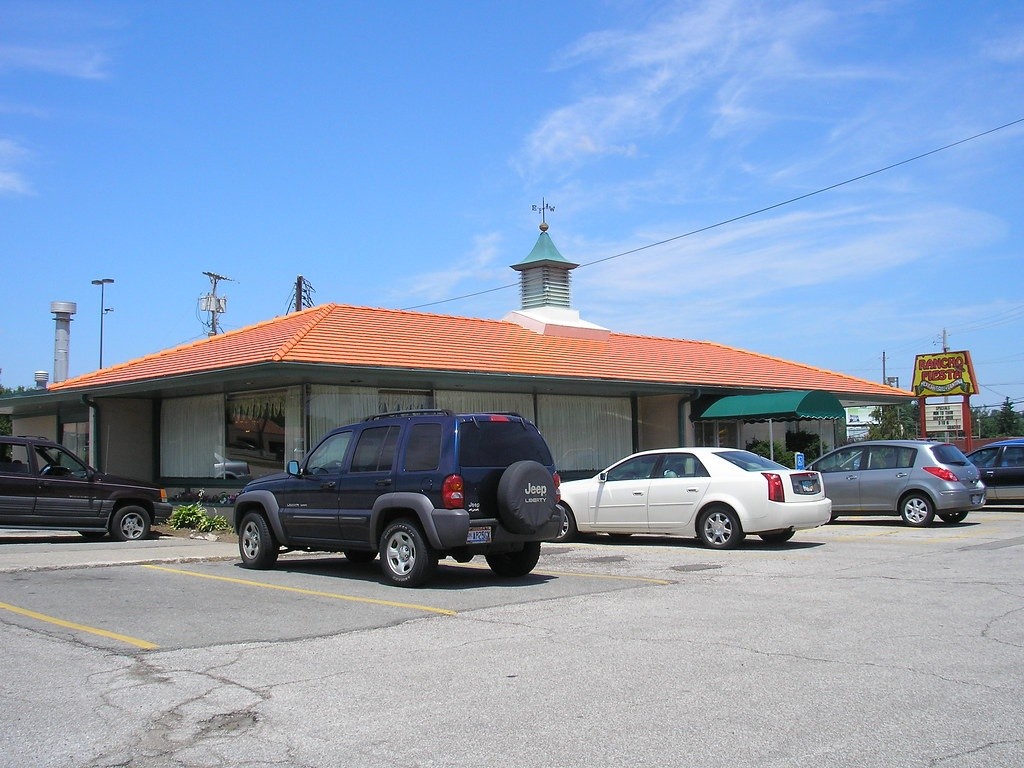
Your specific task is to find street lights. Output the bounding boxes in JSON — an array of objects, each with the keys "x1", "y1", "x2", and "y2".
[{"x1": 92, "y1": 278, "x2": 115, "y2": 369}]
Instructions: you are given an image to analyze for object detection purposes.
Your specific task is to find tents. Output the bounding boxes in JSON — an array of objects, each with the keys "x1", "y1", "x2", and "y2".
[{"x1": 688, "y1": 389, "x2": 847, "y2": 465}]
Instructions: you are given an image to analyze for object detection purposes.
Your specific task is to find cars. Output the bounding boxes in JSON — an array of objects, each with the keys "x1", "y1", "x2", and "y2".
[
  {"x1": 803, "y1": 440, "x2": 987, "y2": 528},
  {"x1": 212, "y1": 452, "x2": 251, "y2": 480},
  {"x1": 959, "y1": 438, "x2": 1024, "y2": 506},
  {"x1": 551, "y1": 446, "x2": 833, "y2": 551}
]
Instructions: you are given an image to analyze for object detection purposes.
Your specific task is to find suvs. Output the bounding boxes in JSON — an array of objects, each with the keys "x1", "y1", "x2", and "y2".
[
  {"x1": 233, "y1": 408, "x2": 565, "y2": 588},
  {"x1": 0, "y1": 435, "x2": 173, "y2": 542}
]
